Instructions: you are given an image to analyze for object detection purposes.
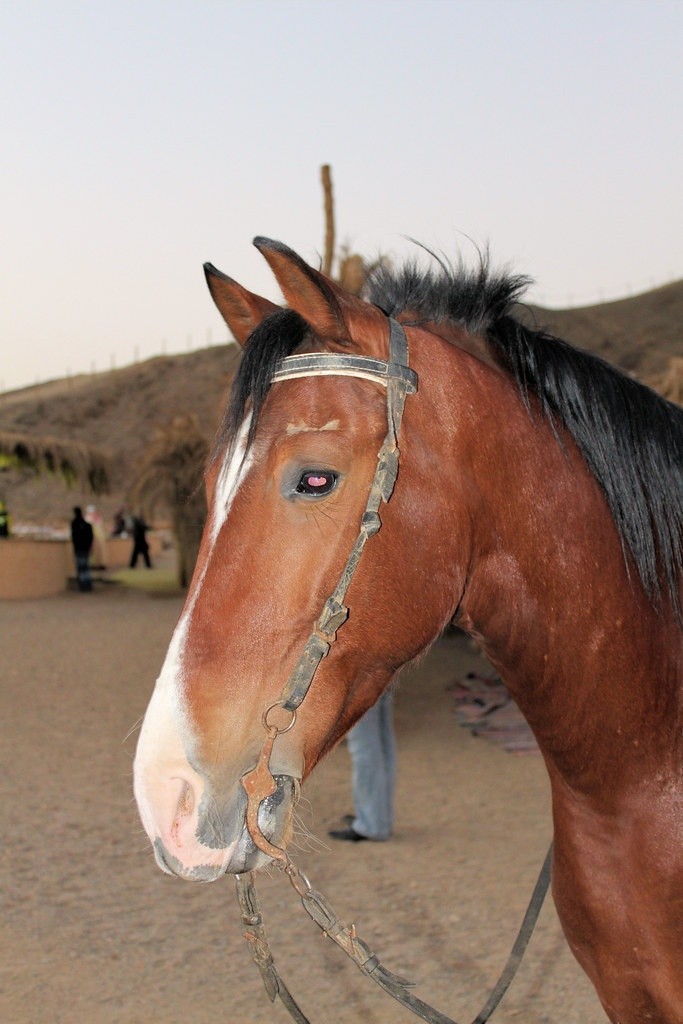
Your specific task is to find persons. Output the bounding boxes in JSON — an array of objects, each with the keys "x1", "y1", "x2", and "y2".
[{"x1": 71, "y1": 494, "x2": 176, "y2": 592}]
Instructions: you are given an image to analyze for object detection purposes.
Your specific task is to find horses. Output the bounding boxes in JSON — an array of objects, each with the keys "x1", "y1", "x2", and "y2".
[{"x1": 131, "y1": 235, "x2": 683, "y2": 1023}]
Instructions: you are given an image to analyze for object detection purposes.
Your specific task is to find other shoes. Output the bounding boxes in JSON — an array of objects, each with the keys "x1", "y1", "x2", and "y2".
[{"x1": 329, "y1": 815, "x2": 367, "y2": 841}]
